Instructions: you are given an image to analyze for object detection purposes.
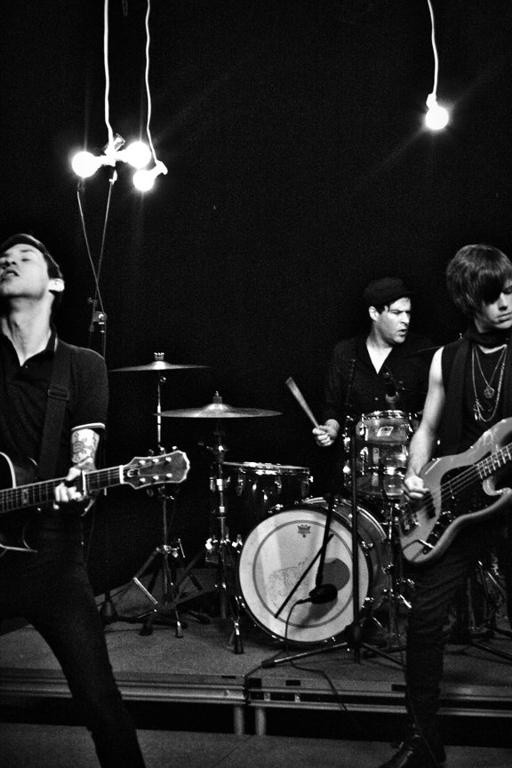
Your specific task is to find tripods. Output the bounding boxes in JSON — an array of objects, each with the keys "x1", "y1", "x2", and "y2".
[
  {"x1": 260, "y1": 360, "x2": 405, "y2": 669},
  {"x1": 361, "y1": 496, "x2": 412, "y2": 647},
  {"x1": 114, "y1": 377, "x2": 206, "y2": 638},
  {"x1": 443, "y1": 558, "x2": 512, "y2": 665},
  {"x1": 141, "y1": 420, "x2": 253, "y2": 654}
]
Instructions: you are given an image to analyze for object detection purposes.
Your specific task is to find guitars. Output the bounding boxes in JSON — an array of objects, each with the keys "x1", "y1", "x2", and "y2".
[
  {"x1": 397, "y1": 417, "x2": 512, "y2": 564},
  {"x1": 1, "y1": 448, "x2": 192, "y2": 558}
]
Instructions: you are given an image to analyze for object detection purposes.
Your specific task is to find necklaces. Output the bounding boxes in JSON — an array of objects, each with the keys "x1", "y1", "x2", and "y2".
[
  {"x1": 467, "y1": 340, "x2": 509, "y2": 424},
  {"x1": 475, "y1": 346, "x2": 506, "y2": 400}
]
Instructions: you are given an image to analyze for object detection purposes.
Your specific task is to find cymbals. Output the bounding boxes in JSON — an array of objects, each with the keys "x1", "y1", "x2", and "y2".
[
  {"x1": 153, "y1": 404, "x2": 283, "y2": 417},
  {"x1": 109, "y1": 361, "x2": 211, "y2": 371}
]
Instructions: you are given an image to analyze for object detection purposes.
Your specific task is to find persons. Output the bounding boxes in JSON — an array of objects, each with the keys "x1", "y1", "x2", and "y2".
[
  {"x1": 378, "y1": 243, "x2": 512, "y2": 768},
  {"x1": 311, "y1": 277, "x2": 429, "y2": 490},
  {"x1": 0, "y1": 233, "x2": 148, "y2": 768}
]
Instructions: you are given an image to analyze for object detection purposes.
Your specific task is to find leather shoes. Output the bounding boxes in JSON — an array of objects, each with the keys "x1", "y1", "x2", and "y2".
[{"x1": 386, "y1": 738, "x2": 447, "y2": 767}]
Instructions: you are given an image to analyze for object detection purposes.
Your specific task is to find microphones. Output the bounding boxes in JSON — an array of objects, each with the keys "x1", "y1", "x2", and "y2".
[{"x1": 307, "y1": 582, "x2": 340, "y2": 606}]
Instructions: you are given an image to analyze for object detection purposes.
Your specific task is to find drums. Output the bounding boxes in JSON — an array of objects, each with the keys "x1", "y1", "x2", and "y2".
[
  {"x1": 237, "y1": 499, "x2": 393, "y2": 647},
  {"x1": 359, "y1": 409, "x2": 414, "y2": 447},
  {"x1": 343, "y1": 451, "x2": 408, "y2": 497},
  {"x1": 221, "y1": 461, "x2": 312, "y2": 520}
]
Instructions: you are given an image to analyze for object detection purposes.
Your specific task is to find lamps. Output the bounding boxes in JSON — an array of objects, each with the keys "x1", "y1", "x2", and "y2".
[
  {"x1": 132, "y1": 0, "x2": 169, "y2": 192},
  {"x1": 423, "y1": 0, "x2": 449, "y2": 134},
  {"x1": 72, "y1": 0, "x2": 151, "y2": 179}
]
